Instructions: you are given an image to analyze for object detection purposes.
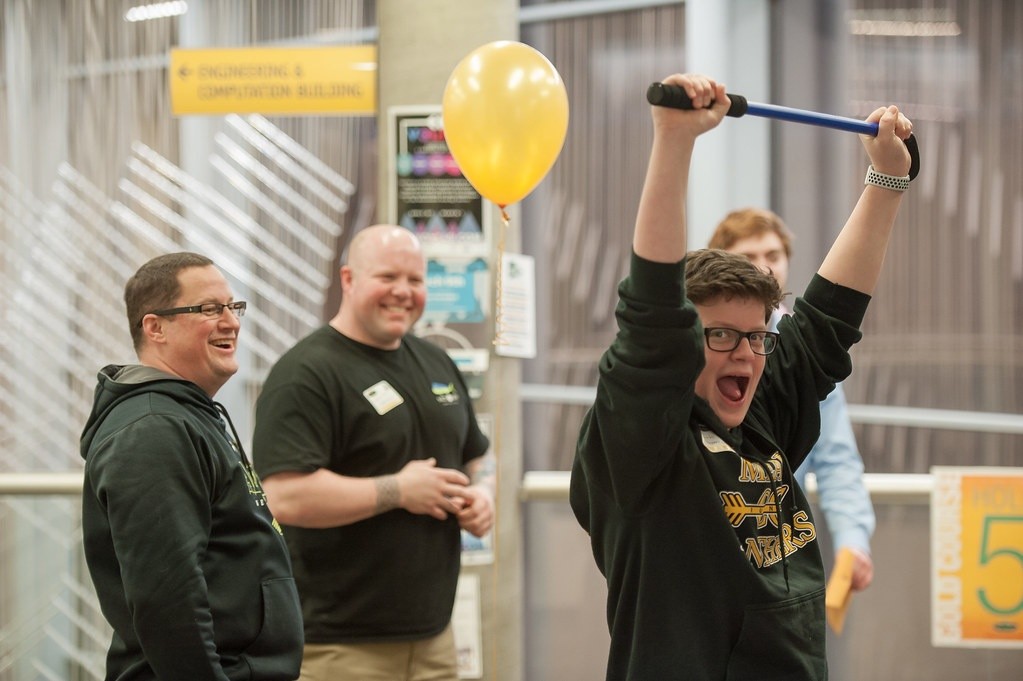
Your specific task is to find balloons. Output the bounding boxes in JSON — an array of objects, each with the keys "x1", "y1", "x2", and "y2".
[{"x1": 442, "y1": 40, "x2": 569, "y2": 226}]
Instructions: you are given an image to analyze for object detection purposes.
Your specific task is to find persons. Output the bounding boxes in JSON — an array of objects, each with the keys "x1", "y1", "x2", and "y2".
[
  {"x1": 701, "y1": 204, "x2": 875, "y2": 591},
  {"x1": 569, "y1": 71, "x2": 912, "y2": 681},
  {"x1": 251, "y1": 222, "x2": 497, "y2": 681},
  {"x1": 81, "y1": 252, "x2": 305, "y2": 681}
]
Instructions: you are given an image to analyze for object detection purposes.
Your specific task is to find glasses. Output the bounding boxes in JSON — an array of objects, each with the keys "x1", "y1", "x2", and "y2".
[
  {"x1": 136, "y1": 301, "x2": 247, "y2": 327},
  {"x1": 704, "y1": 327, "x2": 781, "y2": 355}
]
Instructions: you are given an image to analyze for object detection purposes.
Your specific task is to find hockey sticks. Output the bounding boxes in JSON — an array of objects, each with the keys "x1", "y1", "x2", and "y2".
[{"x1": 645, "y1": 79, "x2": 920, "y2": 180}]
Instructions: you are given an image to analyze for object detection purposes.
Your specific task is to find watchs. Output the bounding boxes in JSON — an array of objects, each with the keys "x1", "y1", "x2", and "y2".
[{"x1": 864, "y1": 166, "x2": 910, "y2": 191}]
